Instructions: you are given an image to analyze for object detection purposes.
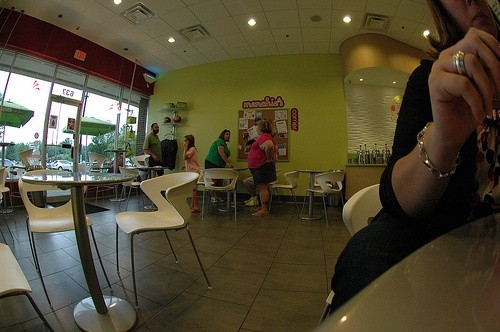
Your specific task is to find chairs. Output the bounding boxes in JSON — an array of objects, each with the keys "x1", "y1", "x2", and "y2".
[
  {"x1": 0, "y1": 148, "x2": 212, "y2": 332},
  {"x1": 251, "y1": 169, "x2": 383, "y2": 324},
  {"x1": 196, "y1": 169, "x2": 239, "y2": 222}
]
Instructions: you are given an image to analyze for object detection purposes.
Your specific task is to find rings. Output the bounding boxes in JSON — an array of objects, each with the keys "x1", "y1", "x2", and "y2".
[{"x1": 452, "y1": 49, "x2": 468, "y2": 77}]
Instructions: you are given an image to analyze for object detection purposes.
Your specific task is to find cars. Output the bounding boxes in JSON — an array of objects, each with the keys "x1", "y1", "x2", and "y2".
[{"x1": 0, "y1": 159, "x2": 72, "y2": 171}]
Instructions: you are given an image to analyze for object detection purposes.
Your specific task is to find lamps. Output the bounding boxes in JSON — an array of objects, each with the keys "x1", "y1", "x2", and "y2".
[{"x1": 143, "y1": 73, "x2": 155, "y2": 83}]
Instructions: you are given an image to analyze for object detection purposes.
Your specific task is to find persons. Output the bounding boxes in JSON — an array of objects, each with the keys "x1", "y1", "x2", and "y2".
[
  {"x1": 318, "y1": 0, "x2": 500, "y2": 324},
  {"x1": 248, "y1": 119, "x2": 278, "y2": 216},
  {"x1": 142, "y1": 123, "x2": 164, "y2": 177},
  {"x1": 205, "y1": 129, "x2": 231, "y2": 203},
  {"x1": 180, "y1": 135, "x2": 201, "y2": 213},
  {"x1": 244, "y1": 140, "x2": 259, "y2": 206}
]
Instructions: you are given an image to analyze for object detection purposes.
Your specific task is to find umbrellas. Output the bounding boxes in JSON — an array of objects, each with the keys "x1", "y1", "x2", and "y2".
[
  {"x1": 62, "y1": 117, "x2": 117, "y2": 163},
  {"x1": 0, "y1": 98, "x2": 34, "y2": 143}
]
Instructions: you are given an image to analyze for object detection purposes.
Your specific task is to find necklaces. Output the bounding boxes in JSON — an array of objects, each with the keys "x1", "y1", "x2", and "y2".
[{"x1": 417, "y1": 122, "x2": 462, "y2": 178}]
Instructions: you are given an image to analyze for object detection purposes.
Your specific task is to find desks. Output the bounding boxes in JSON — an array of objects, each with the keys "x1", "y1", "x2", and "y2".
[
  {"x1": 0, "y1": 142, "x2": 17, "y2": 214},
  {"x1": 104, "y1": 148, "x2": 128, "y2": 202},
  {"x1": 345, "y1": 163, "x2": 387, "y2": 201},
  {"x1": 218, "y1": 168, "x2": 249, "y2": 212},
  {"x1": 135, "y1": 166, "x2": 169, "y2": 211},
  {"x1": 294, "y1": 169, "x2": 323, "y2": 221},
  {"x1": 20, "y1": 170, "x2": 140, "y2": 332}
]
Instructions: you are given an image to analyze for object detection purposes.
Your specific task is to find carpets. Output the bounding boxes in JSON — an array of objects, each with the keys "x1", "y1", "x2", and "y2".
[{"x1": 48, "y1": 199, "x2": 110, "y2": 214}]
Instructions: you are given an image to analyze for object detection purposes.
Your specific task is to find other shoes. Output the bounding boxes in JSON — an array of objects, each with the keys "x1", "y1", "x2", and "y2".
[
  {"x1": 191, "y1": 208, "x2": 200, "y2": 213},
  {"x1": 251, "y1": 207, "x2": 270, "y2": 217},
  {"x1": 244, "y1": 198, "x2": 259, "y2": 206}
]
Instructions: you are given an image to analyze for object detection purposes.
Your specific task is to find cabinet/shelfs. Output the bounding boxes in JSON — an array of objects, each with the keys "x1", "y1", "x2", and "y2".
[{"x1": 158, "y1": 107, "x2": 185, "y2": 136}]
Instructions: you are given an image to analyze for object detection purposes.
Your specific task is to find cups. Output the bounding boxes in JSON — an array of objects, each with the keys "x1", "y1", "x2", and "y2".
[{"x1": 348, "y1": 159, "x2": 352, "y2": 163}]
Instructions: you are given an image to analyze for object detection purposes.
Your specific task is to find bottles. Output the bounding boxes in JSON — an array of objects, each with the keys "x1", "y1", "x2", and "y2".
[
  {"x1": 353, "y1": 155, "x2": 358, "y2": 164},
  {"x1": 357, "y1": 143, "x2": 390, "y2": 164}
]
